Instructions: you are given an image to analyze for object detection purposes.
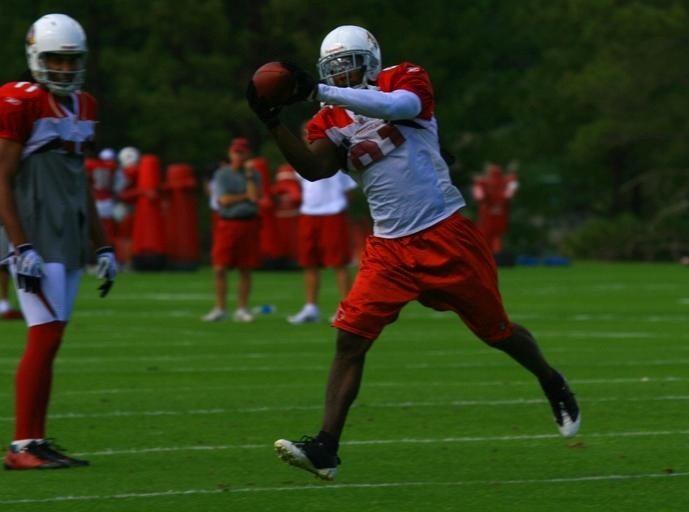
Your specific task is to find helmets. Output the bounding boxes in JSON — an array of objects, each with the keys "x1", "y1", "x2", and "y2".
[
  {"x1": 317, "y1": 25, "x2": 381, "y2": 88},
  {"x1": 25, "y1": 13, "x2": 87, "y2": 95}
]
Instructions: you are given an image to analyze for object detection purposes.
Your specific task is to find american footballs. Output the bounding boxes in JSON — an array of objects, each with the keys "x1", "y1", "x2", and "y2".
[{"x1": 251, "y1": 61, "x2": 297, "y2": 103}]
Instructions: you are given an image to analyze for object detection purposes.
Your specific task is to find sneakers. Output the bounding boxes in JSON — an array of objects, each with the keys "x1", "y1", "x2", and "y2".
[
  {"x1": 274, "y1": 436, "x2": 341, "y2": 481},
  {"x1": 545, "y1": 371, "x2": 580, "y2": 437},
  {"x1": 288, "y1": 308, "x2": 320, "y2": 324},
  {"x1": 4, "y1": 439, "x2": 89, "y2": 469},
  {"x1": 205, "y1": 308, "x2": 253, "y2": 321}
]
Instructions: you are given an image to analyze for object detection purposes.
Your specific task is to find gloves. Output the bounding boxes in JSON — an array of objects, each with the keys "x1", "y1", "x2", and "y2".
[
  {"x1": 96, "y1": 246, "x2": 117, "y2": 296},
  {"x1": 15, "y1": 243, "x2": 48, "y2": 293}
]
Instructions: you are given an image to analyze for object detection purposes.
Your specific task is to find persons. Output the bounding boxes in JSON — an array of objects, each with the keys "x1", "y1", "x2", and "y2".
[
  {"x1": 199, "y1": 137, "x2": 262, "y2": 323},
  {"x1": 1, "y1": 225, "x2": 22, "y2": 320},
  {"x1": 467, "y1": 163, "x2": 519, "y2": 258},
  {"x1": 251, "y1": 156, "x2": 359, "y2": 328},
  {"x1": 247, "y1": 24, "x2": 583, "y2": 480},
  {"x1": 85, "y1": 147, "x2": 202, "y2": 270},
  {"x1": 0, "y1": 13, "x2": 119, "y2": 470}
]
